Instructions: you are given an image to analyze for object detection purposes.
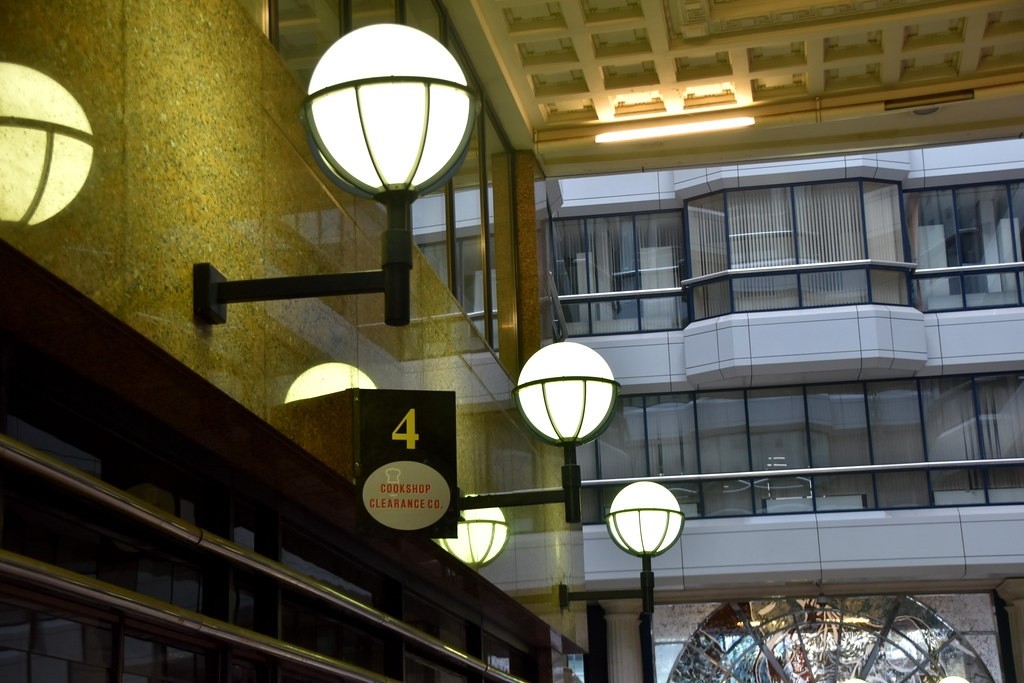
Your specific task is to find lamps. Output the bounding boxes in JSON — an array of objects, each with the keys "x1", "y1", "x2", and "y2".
[
  {"x1": 458, "y1": 341, "x2": 621, "y2": 523},
  {"x1": 440, "y1": 493, "x2": 510, "y2": 567},
  {"x1": 193, "y1": 24, "x2": 486, "y2": 327},
  {"x1": 0, "y1": 62, "x2": 95, "y2": 227},
  {"x1": 285, "y1": 362, "x2": 376, "y2": 402},
  {"x1": 885, "y1": 89, "x2": 975, "y2": 111},
  {"x1": 557, "y1": 481, "x2": 699, "y2": 620}
]
